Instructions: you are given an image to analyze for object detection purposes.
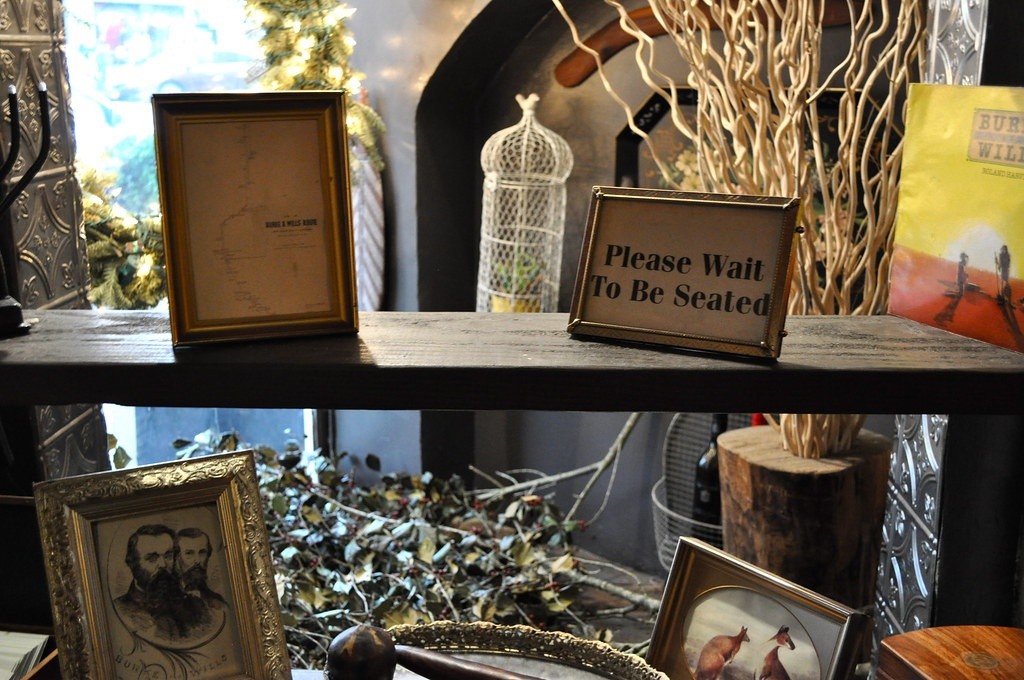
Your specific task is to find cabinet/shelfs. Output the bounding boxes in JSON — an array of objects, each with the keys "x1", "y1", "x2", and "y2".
[{"x1": 0, "y1": 309, "x2": 1024, "y2": 680}]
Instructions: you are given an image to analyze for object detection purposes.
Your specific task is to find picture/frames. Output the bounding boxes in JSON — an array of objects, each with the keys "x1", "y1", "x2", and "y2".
[
  {"x1": 32, "y1": 448, "x2": 293, "y2": 680},
  {"x1": 150, "y1": 89, "x2": 359, "y2": 347},
  {"x1": 646, "y1": 535, "x2": 868, "y2": 679},
  {"x1": 568, "y1": 185, "x2": 804, "y2": 366}
]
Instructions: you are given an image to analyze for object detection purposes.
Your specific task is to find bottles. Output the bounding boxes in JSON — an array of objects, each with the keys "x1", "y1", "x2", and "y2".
[
  {"x1": 692, "y1": 414, "x2": 729, "y2": 550},
  {"x1": 752, "y1": 413, "x2": 766, "y2": 426}
]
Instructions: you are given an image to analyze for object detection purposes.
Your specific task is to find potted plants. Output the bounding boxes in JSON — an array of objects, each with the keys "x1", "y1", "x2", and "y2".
[{"x1": 487, "y1": 253, "x2": 547, "y2": 313}]
